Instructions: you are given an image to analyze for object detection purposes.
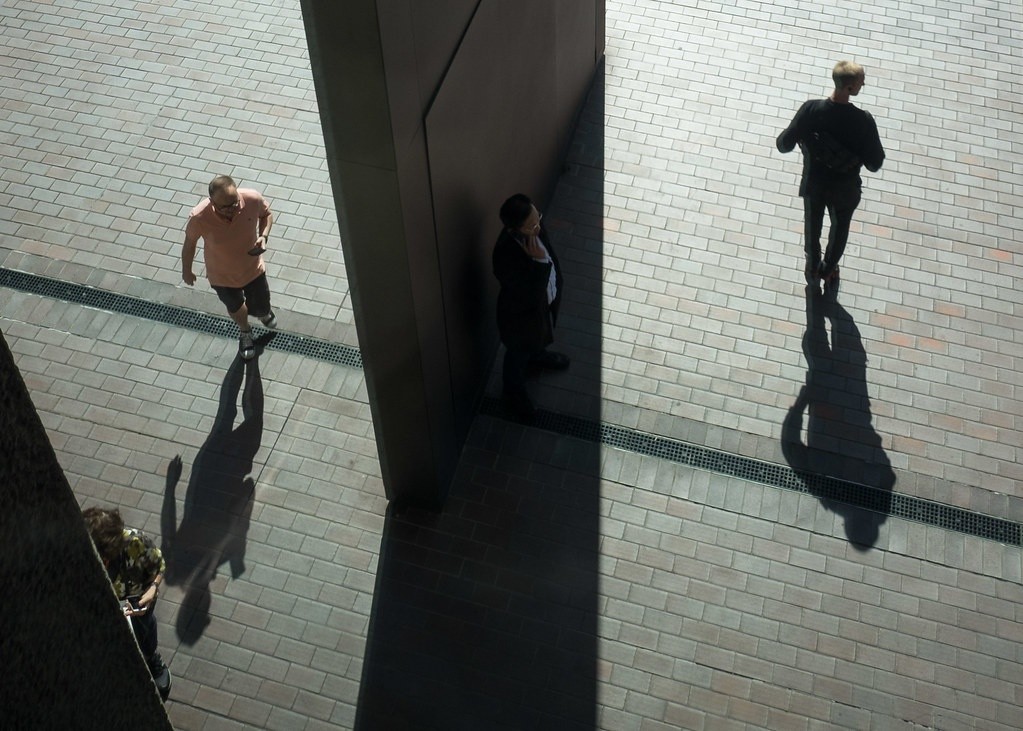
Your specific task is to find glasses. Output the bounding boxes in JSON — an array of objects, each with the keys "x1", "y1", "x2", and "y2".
[
  {"x1": 211, "y1": 197, "x2": 241, "y2": 213},
  {"x1": 518, "y1": 212, "x2": 543, "y2": 236}
]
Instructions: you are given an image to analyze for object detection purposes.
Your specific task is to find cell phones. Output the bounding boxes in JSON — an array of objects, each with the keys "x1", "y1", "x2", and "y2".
[
  {"x1": 248, "y1": 248, "x2": 267, "y2": 256},
  {"x1": 511, "y1": 230, "x2": 529, "y2": 245}
]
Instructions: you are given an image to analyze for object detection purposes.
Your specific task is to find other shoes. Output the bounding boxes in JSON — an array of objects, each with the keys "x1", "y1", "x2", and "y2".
[
  {"x1": 819, "y1": 261, "x2": 840, "y2": 281},
  {"x1": 504, "y1": 401, "x2": 544, "y2": 421},
  {"x1": 535, "y1": 352, "x2": 571, "y2": 371},
  {"x1": 807, "y1": 256, "x2": 822, "y2": 272}
]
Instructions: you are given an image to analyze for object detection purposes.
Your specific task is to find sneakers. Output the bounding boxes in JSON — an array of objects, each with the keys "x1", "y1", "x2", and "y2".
[
  {"x1": 146, "y1": 653, "x2": 172, "y2": 694},
  {"x1": 258, "y1": 310, "x2": 278, "y2": 328},
  {"x1": 239, "y1": 327, "x2": 255, "y2": 360}
]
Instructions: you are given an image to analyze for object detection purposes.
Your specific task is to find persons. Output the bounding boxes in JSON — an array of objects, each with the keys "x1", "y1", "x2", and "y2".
[
  {"x1": 83, "y1": 507, "x2": 172, "y2": 693},
  {"x1": 491, "y1": 192, "x2": 569, "y2": 418},
  {"x1": 776, "y1": 61, "x2": 886, "y2": 279},
  {"x1": 181, "y1": 176, "x2": 277, "y2": 360}
]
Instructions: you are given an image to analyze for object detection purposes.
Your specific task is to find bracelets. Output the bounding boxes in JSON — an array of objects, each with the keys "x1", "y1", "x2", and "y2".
[
  {"x1": 259, "y1": 235, "x2": 268, "y2": 244},
  {"x1": 150, "y1": 582, "x2": 159, "y2": 592}
]
